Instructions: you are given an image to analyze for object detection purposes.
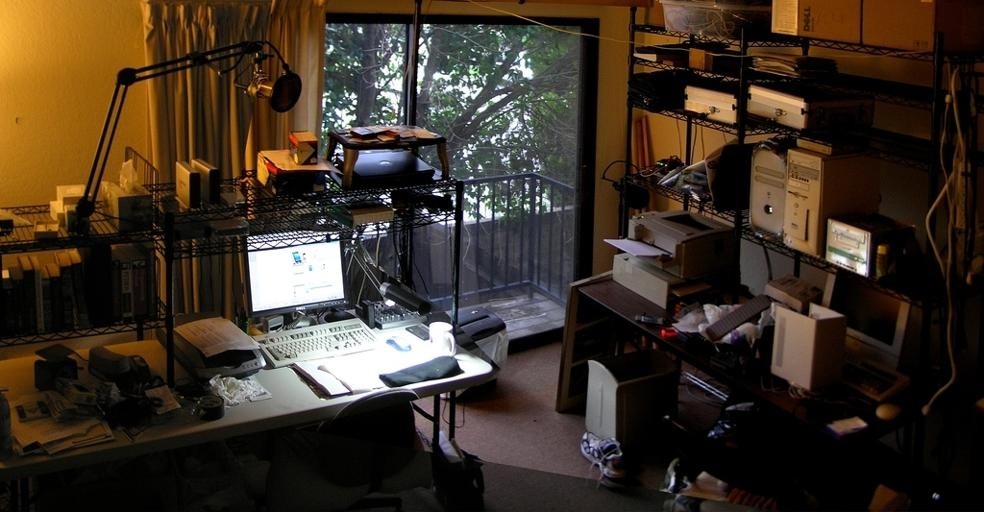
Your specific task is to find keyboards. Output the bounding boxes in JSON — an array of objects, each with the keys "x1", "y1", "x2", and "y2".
[{"x1": 252, "y1": 318, "x2": 385, "y2": 369}]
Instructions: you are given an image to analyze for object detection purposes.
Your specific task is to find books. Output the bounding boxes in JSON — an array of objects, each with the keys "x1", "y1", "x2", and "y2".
[
  {"x1": 0, "y1": 241, "x2": 157, "y2": 336},
  {"x1": 749, "y1": 52, "x2": 838, "y2": 79}
]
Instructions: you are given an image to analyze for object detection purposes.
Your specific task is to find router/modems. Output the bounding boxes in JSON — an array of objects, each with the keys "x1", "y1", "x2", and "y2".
[{"x1": 362, "y1": 300, "x2": 375, "y2": 328}]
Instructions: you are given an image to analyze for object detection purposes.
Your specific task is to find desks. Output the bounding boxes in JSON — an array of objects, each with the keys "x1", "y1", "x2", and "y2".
[
  {"x1": 577, "y1": 279, "x2": 872, "y2": 475},
  {"x1": 0, "y1": 305, "x2": 493, "y2": 512}
]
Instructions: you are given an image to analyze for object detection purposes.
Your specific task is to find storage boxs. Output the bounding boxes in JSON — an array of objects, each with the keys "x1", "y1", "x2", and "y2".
[
  {"x1": 863, "y1": 0, "x2": 984, "y2": 55},
  {"x1": 798, "y1": 0, "x2": 862, "y2": 45}
]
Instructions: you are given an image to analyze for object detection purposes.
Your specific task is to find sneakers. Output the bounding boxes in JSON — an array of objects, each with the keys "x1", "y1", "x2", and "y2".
[{"x1": 581, "y1": 431, "x2": 627, "y2": 478}]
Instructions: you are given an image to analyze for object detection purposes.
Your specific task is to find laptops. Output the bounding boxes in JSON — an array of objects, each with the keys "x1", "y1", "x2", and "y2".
[
  {"x1": 820, "y1": 272, "x2": 911, "y2": 385},
  {"x1": 336, "y1": 151, "x2": 435, "y2": 184}
]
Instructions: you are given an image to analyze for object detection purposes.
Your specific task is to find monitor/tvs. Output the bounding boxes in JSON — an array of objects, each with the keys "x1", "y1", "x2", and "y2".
[{"x1": 242, "y1": 223, "x2": 349, "y2": 331}]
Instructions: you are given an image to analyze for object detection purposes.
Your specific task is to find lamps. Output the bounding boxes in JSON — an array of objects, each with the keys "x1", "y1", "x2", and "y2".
[{"x1": 74, "y1": 40, "x2": 301, "y2": 233}]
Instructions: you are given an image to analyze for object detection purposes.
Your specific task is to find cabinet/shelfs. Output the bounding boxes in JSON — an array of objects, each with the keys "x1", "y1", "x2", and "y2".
[{"x1": 618, "y1": 5, "x2": 984, "y2": 511}]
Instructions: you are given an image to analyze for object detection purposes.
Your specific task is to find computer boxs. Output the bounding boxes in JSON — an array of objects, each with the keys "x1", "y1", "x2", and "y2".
[
  {"x1": 783, "y1": 146, "x2": 881, "y2": 259},
  {"x1": 748, "y1": 143, "x2": 787, "y2": 241}
]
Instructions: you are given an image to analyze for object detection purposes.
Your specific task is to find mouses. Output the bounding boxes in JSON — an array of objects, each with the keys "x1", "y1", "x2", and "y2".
[
  {"x1": 875, "y1": 403, "x2": 896, "y2": 420},
  {"x1": 385, "y1": 335, "x2": 412, "y2": 352}
]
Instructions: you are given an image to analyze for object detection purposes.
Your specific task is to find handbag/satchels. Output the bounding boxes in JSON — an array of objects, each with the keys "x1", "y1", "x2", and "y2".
[{"x1": 432, "y1": 442, "x2": 485, "y2": 511}]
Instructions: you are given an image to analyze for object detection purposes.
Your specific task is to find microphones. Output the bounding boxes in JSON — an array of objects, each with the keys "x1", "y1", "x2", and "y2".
[{"x1": 234, "y1": 79, "x2": 276, "y2": 98}]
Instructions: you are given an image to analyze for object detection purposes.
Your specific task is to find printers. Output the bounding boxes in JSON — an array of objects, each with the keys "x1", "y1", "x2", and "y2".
[{"x1": 603, "y1": 210, "x2": 735, "y2": 280}]
